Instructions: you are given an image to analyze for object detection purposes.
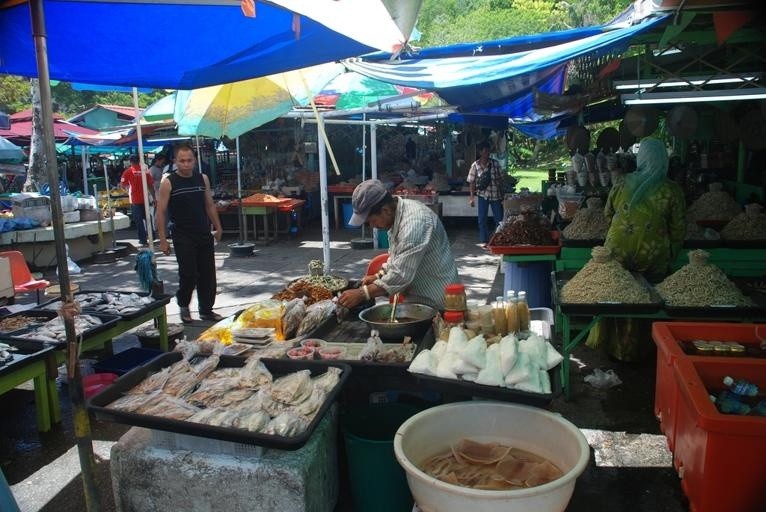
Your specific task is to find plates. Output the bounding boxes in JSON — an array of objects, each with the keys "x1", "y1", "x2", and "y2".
[
  {"x1": 486, "y1": 230, "x2": 561, "y2": 254},
  {"x1": 556, "y1": 223, "x2": 605, "y2": 247},
  {"x1": 87, "y1": 350, "x2": 353, "y2": 451},
  {"x1": 233, "y1": 336, "x2": 275, "y2": 344},
  {"x1": 635, "y1": 270, "x2": 766, "y2": 315},
  {"x1": 408, "y1": 305, "x2": 563, "y2": 401},
  {"x1": 222, "y1": 343, "x2": 253, "y2": 356},
  {"x1": 254, "y1": 342, "x2": 273, "y2": 349},
  {"x1": 240, "y1": 199, "x2": 292, "y2": 206},
  {"x1": 0, "y1": 337, "x2": 57, "y2": 377},
  {"x1": 551, "y1": 270, "x2": 662, "y2": 313},
  {"x1": 32, "y1": 290, "x2": 174, "y2": 320},
  {"x1": 231, "y1": 328, "x2": 276, "y2": 338},
  {"x1": 0, "y1": 310, "x2": 121, "y2": 350}
]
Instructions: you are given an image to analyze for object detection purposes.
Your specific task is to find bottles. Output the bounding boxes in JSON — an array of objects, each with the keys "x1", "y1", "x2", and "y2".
[
  {"x1": 480, "y1": 324, "x2": 492, "y2": 334},
  {"x1": 506, "y1": 300, "x2": 520, "y2": 333},
  {"x1": 518, "y1": 291, "x2": 531, "y2": 332},
  {"x1": 730, "y1": 345, "x2": 742, "y2": 357},
  {"x1": 465, "y1": 321, "x2": 479, "y2": 334},
  {"x1": 479, "y1": 305, "x2": 491, "y2": 323},
  {"x1": 444, "y1": 285, "x2": 465, "y2": 311},
  {"x1": 441, "y1": 311, "x2": 463, "y2": 332},
  {"x1": 495, "y1": 297, "x2": 506, "y2": 334},
  {"x1": 723, "y1": 376, "x2": 763, "y2": 399},
  {"x1": 714, "y1": 345, "x2": 728, "y2": 356},
  {"x1": 708, "y1": 394, "x2": 760, "y2": 418},
  {"x1": 504, "y1": 290, "x2": 515, "y2": 300},
  {"x1": 695, "y1": 345, "x2": 713, "y2": 358},
  {"x1": 467, "y1": 302, "x2": 480, "y2": 321}
]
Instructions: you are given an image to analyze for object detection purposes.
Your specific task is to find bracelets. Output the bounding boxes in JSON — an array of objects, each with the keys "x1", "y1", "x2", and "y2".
[{"x1": 359, "y1": 284, "x2": 372, "y2": 301}]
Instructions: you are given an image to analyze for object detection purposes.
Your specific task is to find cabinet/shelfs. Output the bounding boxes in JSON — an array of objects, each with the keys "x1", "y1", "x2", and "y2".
[
  {"x1": 220, "y1": 186, "x2": 386, "y2": 252},
  {"x1": 487, "y1": 221, "x2": 765, "y2": 404},
  {"x1": 0, "y1": 288, "x2": 177, "y2": 436}
]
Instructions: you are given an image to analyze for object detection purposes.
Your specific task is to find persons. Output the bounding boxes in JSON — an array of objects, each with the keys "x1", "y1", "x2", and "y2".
[
  {"x1": 149, "y1": 155, "x2": 166, "y2": 189},
  {"x1": 155, "y1": 143, "x2": 223, "y2": 325},
  {"x1": 602, "y1": 136, "x2": 687, "y2": 270},
  {"x1": 161, "y1": 154, "x2": 177, "y2": 239},
  {"x1": 466, "y1": 143, "x2": 510, "y2": 246},
  {"x1": 120, "y1": 154, "x2": 164, "y2": 244},
  {"x1": 338, "y1": 179, "x2": 465, "y2": 312}
]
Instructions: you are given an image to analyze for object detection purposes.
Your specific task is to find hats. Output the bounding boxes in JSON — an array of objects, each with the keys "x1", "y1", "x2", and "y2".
[{"x1": 347, "y1": 179, "x2": 387, "y2": 226}]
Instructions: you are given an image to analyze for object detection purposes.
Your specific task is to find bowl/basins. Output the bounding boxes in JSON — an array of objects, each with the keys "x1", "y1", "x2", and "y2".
[
  {"x1": 287, "y1": 347, "x2": 315, "y2": 361},
  {"x1": 300, "y1": 338, "x2": 328, "y2": 354},
  {"x1": 392, "y1": 399, "x2": 592, "y2": 511},
  {"x1": 358, "y1": 301, "x2": 436, "y2": 340},
  {"x1": 286, "y1": 274, "x2": 348, "y2": 295},
  {"x1": 319, "y1": 346, "x2": 347, "y2": 360}
]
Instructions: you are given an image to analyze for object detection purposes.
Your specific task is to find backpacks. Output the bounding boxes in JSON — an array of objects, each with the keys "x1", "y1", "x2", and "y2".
[{"x1": 160, "y1": 163, "x2": 177, "y2": 185}]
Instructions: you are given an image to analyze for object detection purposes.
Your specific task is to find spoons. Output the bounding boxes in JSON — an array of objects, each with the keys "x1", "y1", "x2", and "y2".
[{"x1": 386, "y1": 290, "x2": 400, "y2": 326}]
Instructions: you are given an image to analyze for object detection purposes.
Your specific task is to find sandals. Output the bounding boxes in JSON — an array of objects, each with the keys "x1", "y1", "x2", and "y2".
[
  {"x1": 198, "y1": 311, "x2": 223, "y2": 320},
  {"x1": 179, "y1": 306, "x2": 193, "y2": 323}
]
